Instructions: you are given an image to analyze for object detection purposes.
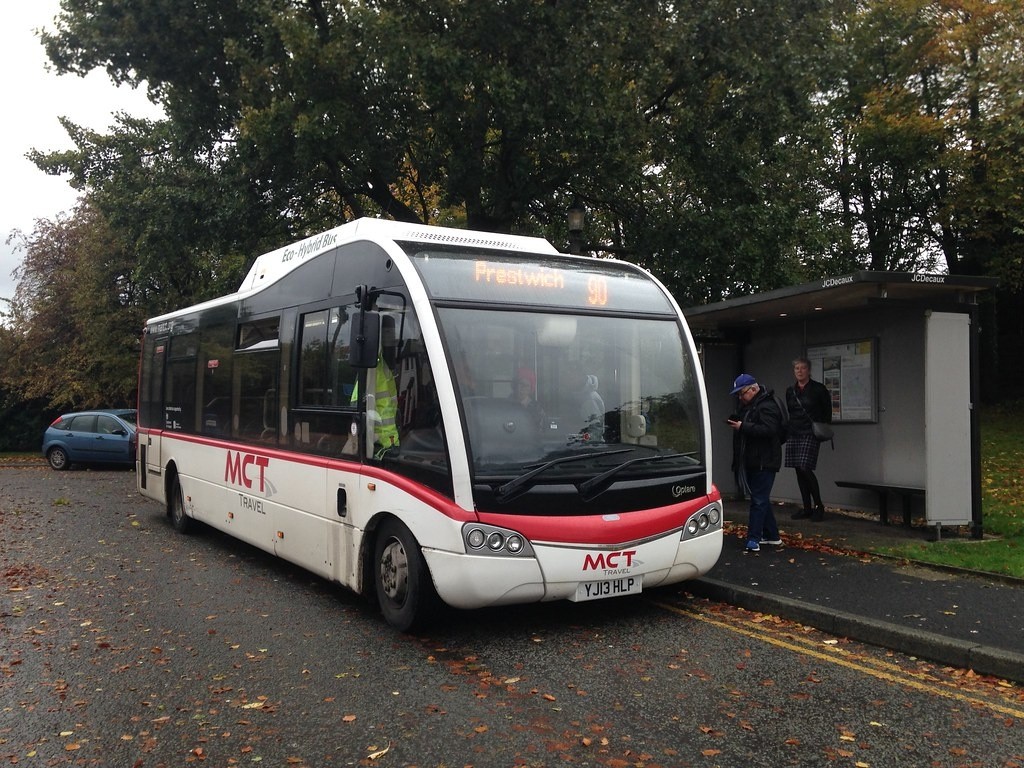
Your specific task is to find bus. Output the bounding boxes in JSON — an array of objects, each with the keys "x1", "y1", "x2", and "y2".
[{"x1": 135, "y1": 216, "x2": 724, "y2": 633}]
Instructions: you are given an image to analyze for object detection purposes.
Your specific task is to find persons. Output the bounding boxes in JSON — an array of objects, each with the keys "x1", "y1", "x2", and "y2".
[
  {"x1": 350, "y1": 345, "x2": 605, "y2": 451},
  {"x1": 784, "y1": 357, "x2": 833, "y2": 523},
  {"x1": 727, "y1": 373, "x2": 782, "y2": 552}
]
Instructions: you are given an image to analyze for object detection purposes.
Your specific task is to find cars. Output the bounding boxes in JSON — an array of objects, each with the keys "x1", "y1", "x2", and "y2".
[{"x1": 41, "y1": 408, "x2": 136, "y2": 471}]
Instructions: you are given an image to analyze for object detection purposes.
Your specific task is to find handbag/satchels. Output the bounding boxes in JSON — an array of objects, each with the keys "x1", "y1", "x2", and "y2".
[{"x1": 811, "y1": 422, "x2": 836, "y2": 452}]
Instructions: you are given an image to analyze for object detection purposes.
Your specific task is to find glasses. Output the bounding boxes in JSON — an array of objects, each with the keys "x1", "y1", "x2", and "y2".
[{"x1": 735, "y1": 386, "x2": 752, "y2": 398}]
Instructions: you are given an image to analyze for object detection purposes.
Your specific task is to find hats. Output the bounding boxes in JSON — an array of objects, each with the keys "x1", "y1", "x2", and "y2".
[{"x1": 729, "y1": 374, "x2": 756, "y2": 395}]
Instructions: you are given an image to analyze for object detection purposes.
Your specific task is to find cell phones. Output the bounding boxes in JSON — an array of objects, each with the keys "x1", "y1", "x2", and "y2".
[{"x1": 728, "y1": 415, "x2": 740, "y2": 424}]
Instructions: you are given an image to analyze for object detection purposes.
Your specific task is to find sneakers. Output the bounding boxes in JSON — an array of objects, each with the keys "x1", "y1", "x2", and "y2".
[
  {"x1": 746, "y1": 540, "x2": 760, "y2": 552},
  {"x1": 759, "y1": 538, "x2": 781, "y2": 545}
]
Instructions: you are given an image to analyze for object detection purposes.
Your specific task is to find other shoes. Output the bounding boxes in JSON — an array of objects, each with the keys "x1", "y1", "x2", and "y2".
[
  {"x1": 812, "y1": 505, "x2": 825, "y2": 521},
  {"x1": 791, "y1": 508, "x2": 812, "y2": 519}
]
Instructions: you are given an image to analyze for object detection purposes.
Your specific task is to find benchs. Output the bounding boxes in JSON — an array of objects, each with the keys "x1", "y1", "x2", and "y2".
[
  {"x1": 834, "y1": 480, "x2": 927, "y2": 530},
  {"x1": 203, "y1": 411, "x2": 335, "y2": 455}
]
